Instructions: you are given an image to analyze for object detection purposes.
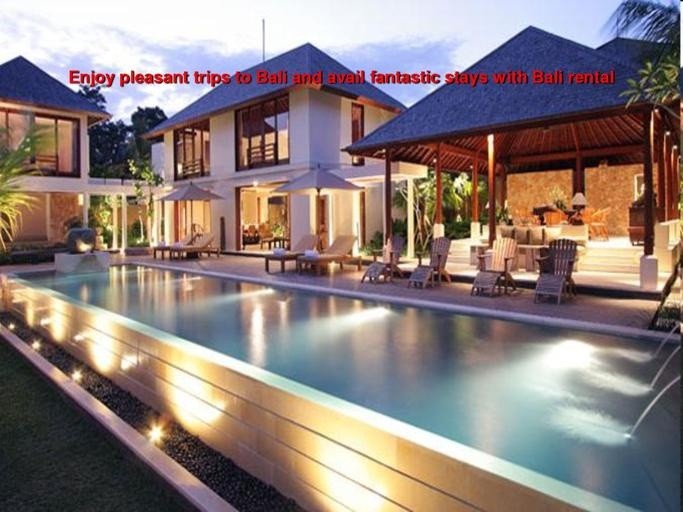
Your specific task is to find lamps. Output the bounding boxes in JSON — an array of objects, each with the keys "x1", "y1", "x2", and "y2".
[{"x1": 570, "y1": 192, "x2": 588, "y2": 225}]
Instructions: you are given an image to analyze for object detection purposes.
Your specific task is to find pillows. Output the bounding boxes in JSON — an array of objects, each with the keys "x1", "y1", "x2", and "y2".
[{"x1": 500, "y1": 226, "x2": 561, "y2": 245}]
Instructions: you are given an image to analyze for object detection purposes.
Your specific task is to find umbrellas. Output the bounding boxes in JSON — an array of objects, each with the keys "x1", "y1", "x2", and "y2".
[
  {"x1": 269, "y1": 159, "x2": 366, "y2": 253},
  {"x1": 152, "y1": 181, "x2": 228, "y2": 243}
]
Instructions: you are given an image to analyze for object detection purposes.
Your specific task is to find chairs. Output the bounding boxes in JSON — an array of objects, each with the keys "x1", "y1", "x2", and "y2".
[
  {"x1": 545, "y1": 206, "x2": 611, "y2": 241},
  {"x1": 242, "y1": 224, "x2": 282, "y2": 250},
  {"x1": 471, "y1": 237, "x2": 518, "y2": 298},
  {"x1": 361, "y1": 235, "x2": 404, "y2": 286},
  {"x1": 534, "y1": 239, "x2": 577, "y2": 305},
  {"x1": 264, "y1": 234, "x2": 361, "y2": 276},
  {"x1": 153, "y1": 232, "x2": 221, "y2": 262},
  {"x1": 407, "y1": 237, "x2": 451, "y2": 289}
]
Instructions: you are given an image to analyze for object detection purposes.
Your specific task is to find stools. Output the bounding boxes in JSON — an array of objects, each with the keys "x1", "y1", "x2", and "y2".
[{"x1": 627, "y1": 226, "x2": 645, "y2": 246}]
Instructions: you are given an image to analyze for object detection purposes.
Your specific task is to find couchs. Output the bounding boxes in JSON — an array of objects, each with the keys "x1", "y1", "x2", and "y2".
[{"x1": 496, "y1": 224, "x2": 588, "y2": 271}]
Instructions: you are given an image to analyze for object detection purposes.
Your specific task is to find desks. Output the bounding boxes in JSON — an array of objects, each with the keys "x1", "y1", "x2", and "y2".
[{"x1": 274, "y1": 237, "x2": 290, "y2": 251}]
